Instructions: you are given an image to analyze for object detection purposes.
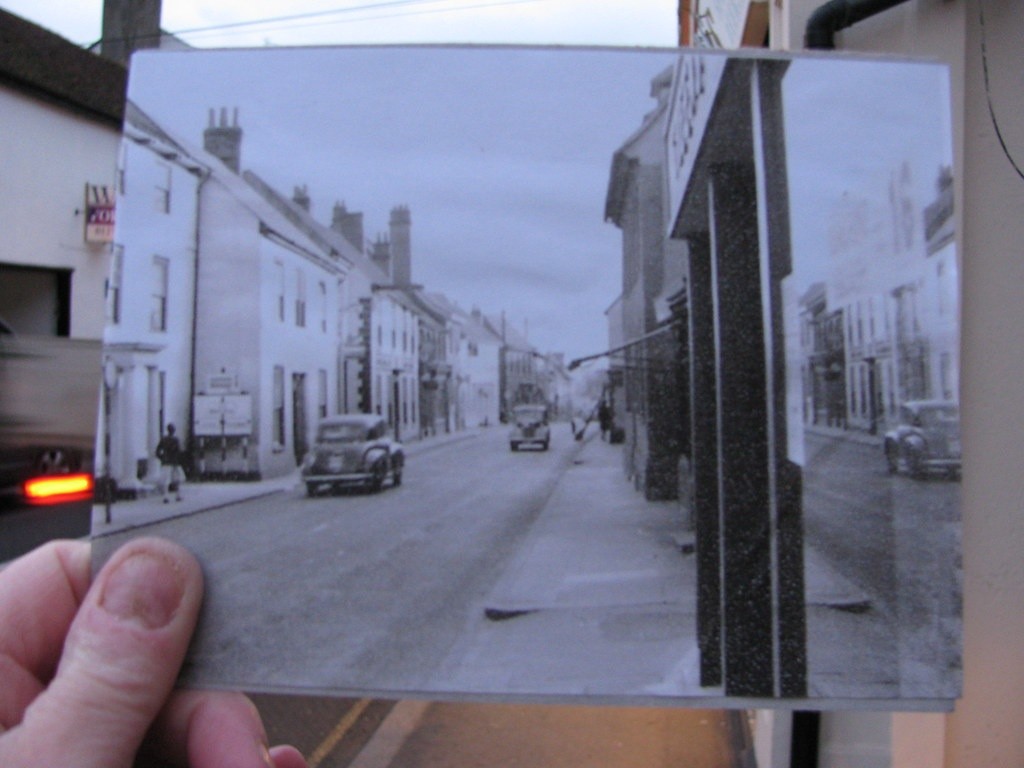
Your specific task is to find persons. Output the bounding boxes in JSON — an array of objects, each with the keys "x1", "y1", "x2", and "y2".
[
  {"x1": 597, "y1": 401, "x2": 615, "y2": 439},
  {"x1": 156, "y1": 424, "x2": 187, "y2": 502}
]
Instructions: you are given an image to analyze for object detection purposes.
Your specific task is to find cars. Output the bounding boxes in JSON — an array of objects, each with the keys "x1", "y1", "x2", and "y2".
[
  {"x1": 508, "y1": 402, "x2": 551, "y2": 451},
  {"x1": 299, "y1": 412, "x2": 403, "y2": 493}
]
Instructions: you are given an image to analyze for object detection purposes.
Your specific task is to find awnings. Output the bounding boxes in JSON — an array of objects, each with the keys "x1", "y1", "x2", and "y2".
[{"x1": 568, "y1": 318, "x2": 688, "y2": 375}]
